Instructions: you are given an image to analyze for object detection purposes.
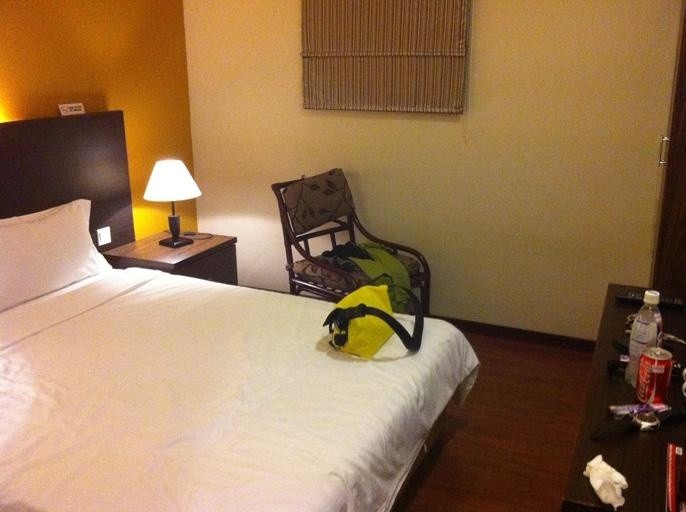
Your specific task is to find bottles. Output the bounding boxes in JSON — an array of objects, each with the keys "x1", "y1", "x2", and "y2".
[{"x1": 624, "y1": 289, "x2": 663, "y2": 386}]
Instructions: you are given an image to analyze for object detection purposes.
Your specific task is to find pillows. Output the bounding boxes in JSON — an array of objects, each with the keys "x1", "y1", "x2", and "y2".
[{"x1": 0, "y1": 198, "x2": 112, "y2": 313}]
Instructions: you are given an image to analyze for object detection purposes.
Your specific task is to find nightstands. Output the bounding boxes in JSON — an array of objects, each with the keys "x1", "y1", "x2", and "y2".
[{"x1": 102, "y1": 229, "x2": 238, "y2": 286}]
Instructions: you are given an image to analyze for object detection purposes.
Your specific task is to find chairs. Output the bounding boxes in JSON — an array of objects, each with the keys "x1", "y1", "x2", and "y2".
[{"x1": 270, "y1": 168, "x2": 431, "y2": 318}]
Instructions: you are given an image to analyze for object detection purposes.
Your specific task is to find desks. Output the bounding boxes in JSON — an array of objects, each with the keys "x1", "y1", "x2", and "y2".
[{"x1": 559, "y1": 281, "x2": 685, "y2": 512}]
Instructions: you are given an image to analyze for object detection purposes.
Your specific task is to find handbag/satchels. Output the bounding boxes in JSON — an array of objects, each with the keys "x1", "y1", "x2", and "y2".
[{"x1": 322, "y1": 283, "x2": 424, "y2": 360}]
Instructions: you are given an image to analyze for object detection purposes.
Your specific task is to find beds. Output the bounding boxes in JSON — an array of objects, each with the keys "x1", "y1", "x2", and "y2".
[{"x1": 1, "y1": 108, "x2": 481, "y2": 512}]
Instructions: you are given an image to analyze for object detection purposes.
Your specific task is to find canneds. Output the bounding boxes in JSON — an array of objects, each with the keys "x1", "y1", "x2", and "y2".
[{"x1": 635, "y1": 347, "x2": 673, "y2": 406}]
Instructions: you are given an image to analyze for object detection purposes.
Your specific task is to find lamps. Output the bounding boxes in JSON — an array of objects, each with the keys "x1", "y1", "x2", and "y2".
[{"x1": 143, "y1": 159, "x2": 201, "y2": 249}]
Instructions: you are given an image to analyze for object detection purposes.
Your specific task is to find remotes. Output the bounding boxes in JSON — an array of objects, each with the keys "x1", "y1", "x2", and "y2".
[{"x1": 615, "y1": 291, "x2": 682, "y2": 308}]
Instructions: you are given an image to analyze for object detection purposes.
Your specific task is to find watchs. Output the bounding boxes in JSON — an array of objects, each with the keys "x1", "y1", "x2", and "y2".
[{"x1": 592, "y1": 404, "x2": 683, "y2": 443}]
